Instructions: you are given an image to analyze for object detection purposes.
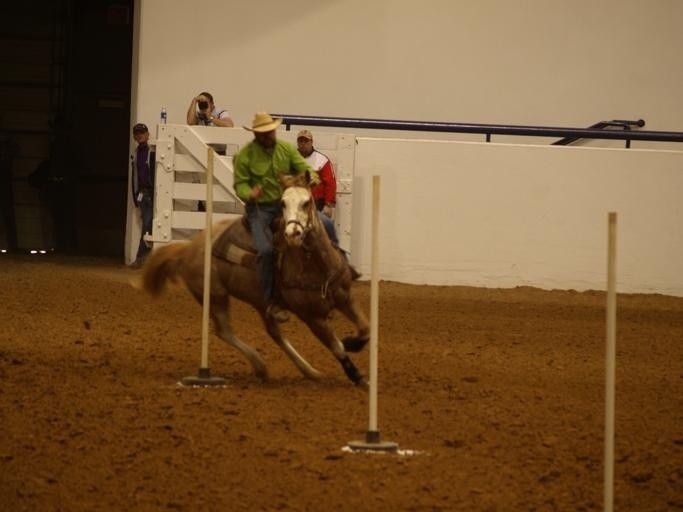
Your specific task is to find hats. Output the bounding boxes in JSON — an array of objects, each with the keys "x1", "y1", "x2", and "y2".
[
  {"x1": 133, "y1": 123, "x2": 149, "y2": 135},
  {"x1": 240, "y1": 111, "x2": 283, "y2": 134},
  {"x1": 297, "y1": 129, "x2": 313, "y2": 141}
]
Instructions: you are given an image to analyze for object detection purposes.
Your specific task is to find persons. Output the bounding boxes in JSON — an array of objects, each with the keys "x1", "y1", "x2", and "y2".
[
  {"x1": 125, "y1": 118, "x2": 157, "y2": 269},
  {"x1": 185, "y1": 90, "x2": 239, "y2": 153},
  {"x1": 288, "y1": 131, "x2": 335, "y2": 228},
  {"x1": 230, "y1": 109, "x2": 319, "y2": 324}
]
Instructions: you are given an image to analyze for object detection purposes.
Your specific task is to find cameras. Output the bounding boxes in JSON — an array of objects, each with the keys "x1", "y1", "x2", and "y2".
[{"x1": 198, "y1": 101, "x2": 208, "y2": 110}]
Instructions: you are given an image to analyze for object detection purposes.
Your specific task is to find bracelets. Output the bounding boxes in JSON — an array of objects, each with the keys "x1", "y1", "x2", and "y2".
[{"x1": 206, "y1": 115, "x2": 215, "y2": 122}]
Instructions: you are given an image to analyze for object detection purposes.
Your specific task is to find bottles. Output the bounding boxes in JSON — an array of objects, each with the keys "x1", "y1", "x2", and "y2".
[{"x1": 161, "y1": 106, "x2": 167, "y2": 125}]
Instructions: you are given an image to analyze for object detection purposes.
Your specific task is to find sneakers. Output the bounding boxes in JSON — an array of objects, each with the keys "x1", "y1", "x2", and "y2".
[
  {"x1": 127, "y1": 253, "x2": 149, "y2": 270},
  {"x1": 265, "y1": 304, "x2": 288, "y2": 322}
]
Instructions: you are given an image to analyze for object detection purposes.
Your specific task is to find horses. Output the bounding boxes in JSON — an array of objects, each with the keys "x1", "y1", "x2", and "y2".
[{"x1": 142, "y1": 168, "x2": 370, "y2": 391}]
